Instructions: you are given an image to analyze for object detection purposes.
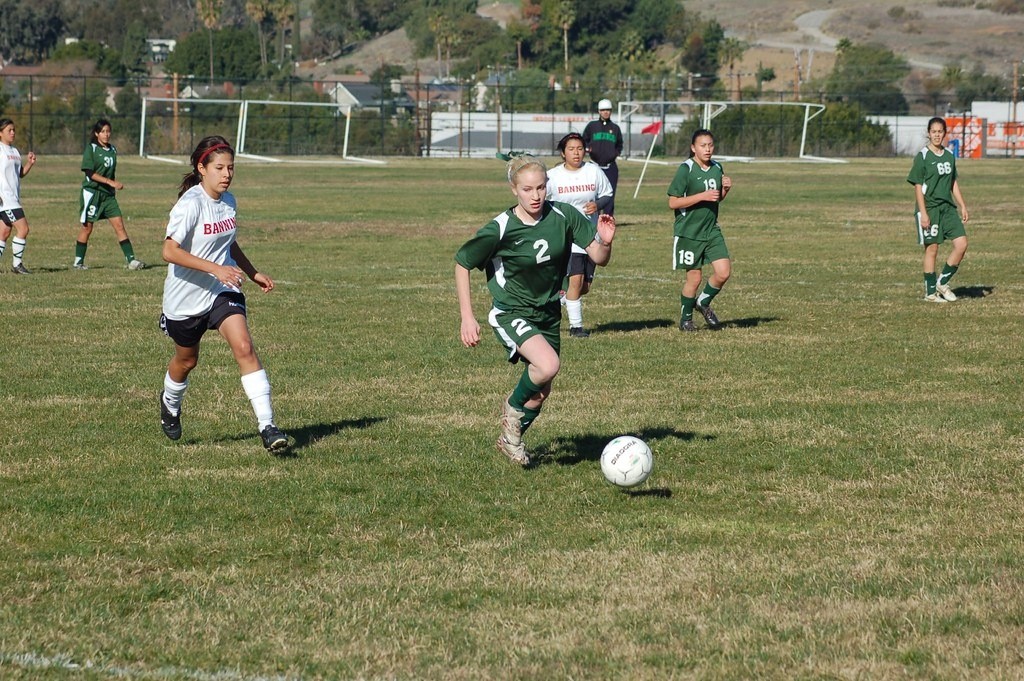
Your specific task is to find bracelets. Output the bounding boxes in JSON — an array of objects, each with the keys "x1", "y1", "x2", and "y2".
[{"x1": 595, "y1": 233, "x2": 610, "y2": 247}]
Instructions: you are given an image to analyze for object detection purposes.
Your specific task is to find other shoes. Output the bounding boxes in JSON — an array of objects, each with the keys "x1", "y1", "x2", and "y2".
[
  {"x1": 11, "y1": 263, "x2": 32, "y2": 275},
  {"x1": 570, "y1": 326, "x2": 589, "y2": 338},
  {"x1": 72, "y1": 265, "x2": 88, "y2": 271},
  {"x1": 125, "y1": 259, "x2": 145, "y2": 270}
]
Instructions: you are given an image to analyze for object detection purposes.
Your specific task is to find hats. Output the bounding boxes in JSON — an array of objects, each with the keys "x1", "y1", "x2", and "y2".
[{"x1": 598, "y1": 99, "x2": 612, "y2": 110}]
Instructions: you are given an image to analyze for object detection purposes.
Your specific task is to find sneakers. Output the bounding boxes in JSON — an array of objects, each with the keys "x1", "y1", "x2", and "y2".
[
  {"x1": 936, "y1": 281, "x2": 957, "y2": 301},
  {"x1": 694, "y1": 298, "x2": 719, "y2": 326},
  {"x1": 680, "y1": 321, "x2": 699, "y2": 332},
  {"x1": 259, "y1": 424, "x2": 288, "y2": 451},
  {"x1": 497, "y1": 434, "x2": 530, "y2": 468},
  {"x1": 500, "y1": 391, "x2": 525, "y2": 446},
  {"x1": 924, "y1": 292, "x2": 948, "y2": 303},
  {"x1": 160, "y1": 390, "x2": 182, "y2": 441}
]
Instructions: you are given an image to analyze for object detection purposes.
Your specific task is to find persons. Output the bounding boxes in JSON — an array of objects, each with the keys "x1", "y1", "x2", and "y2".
[
  {"x1": 545, "y1": 132, "x2": 613, "y2": 338},
  {"x1": 545, "y1": 75, "x2": 556, "y2": 112},
  {"x1": 160, "y1": 135, "x2": 289, "y2": 452},
  {"x1": 73, "y1": 120, "x2": 146, "y2": 271},
  {"x1": 563, "y1": 76, "x2": 580, "y2": 113},
  {"x1": 907, "y1": 118, "x2": 968, "y2": 304},
  {"x1": 455, "y1": 152, "x2": 615, "y2": 463},
  {"x1": 582, "y1": 99, "x2": 623, "y2": 217},
  {"x1": 667, "y1": 129, "x2": 732, "y2": 332},
  {"x1": 0, "y1": 118, "x2": 36, "y2": 274}
]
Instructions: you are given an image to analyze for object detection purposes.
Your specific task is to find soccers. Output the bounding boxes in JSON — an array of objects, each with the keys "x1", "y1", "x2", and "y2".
[{"x1": 599, "y1": 436, "x2": 653, "y2": 489}]
